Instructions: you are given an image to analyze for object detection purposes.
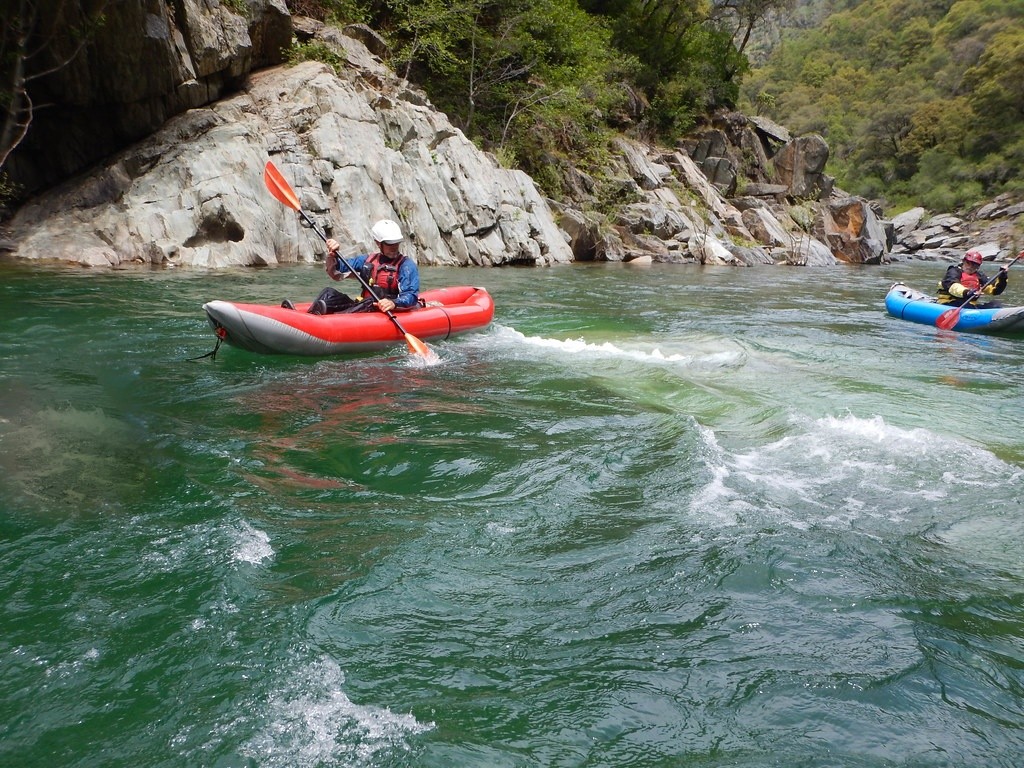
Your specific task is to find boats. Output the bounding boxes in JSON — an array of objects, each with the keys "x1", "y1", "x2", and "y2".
[
  {"x1": 201, "y1": 285, "x2": 494, "y2": 356},
  {"x1": 886, "y1": 281, "x2": 1024, "y2": 333}
]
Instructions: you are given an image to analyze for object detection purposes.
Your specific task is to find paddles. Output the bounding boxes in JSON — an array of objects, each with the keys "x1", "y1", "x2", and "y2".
[
  {"x1": 936, "y1": 247, "x2": 1024, "y2": 331},
  {"x1": 264, "y1": 160, "x2": 435, "y2": 358}
]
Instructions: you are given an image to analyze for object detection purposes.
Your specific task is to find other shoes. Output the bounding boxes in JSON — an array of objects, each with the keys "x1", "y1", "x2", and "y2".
[
  {"x1": 307, "y1": 299, "x2": 327, "y2": 315},
  {"x1": 280, "y1": 299, "x2": 295, "y2": 310}
]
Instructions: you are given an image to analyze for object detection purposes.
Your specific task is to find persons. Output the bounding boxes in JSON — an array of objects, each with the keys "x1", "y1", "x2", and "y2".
[
  {"x1": 280, "y1": 219, "x2": 420, "y2": 315},
  {"x1": 936, "y1": 250, "x2": 1010, "y2": 308}
]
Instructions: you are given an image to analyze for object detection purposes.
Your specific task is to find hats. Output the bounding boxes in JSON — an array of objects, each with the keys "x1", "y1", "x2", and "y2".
[{"x1": 369, "y1": 219, "x2": 405, "y2": 244}]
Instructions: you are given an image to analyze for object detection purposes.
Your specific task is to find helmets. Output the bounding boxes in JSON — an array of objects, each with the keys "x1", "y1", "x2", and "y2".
[{"x1": 964, "y1": 251, "x2": 983, "y2": 265}]
